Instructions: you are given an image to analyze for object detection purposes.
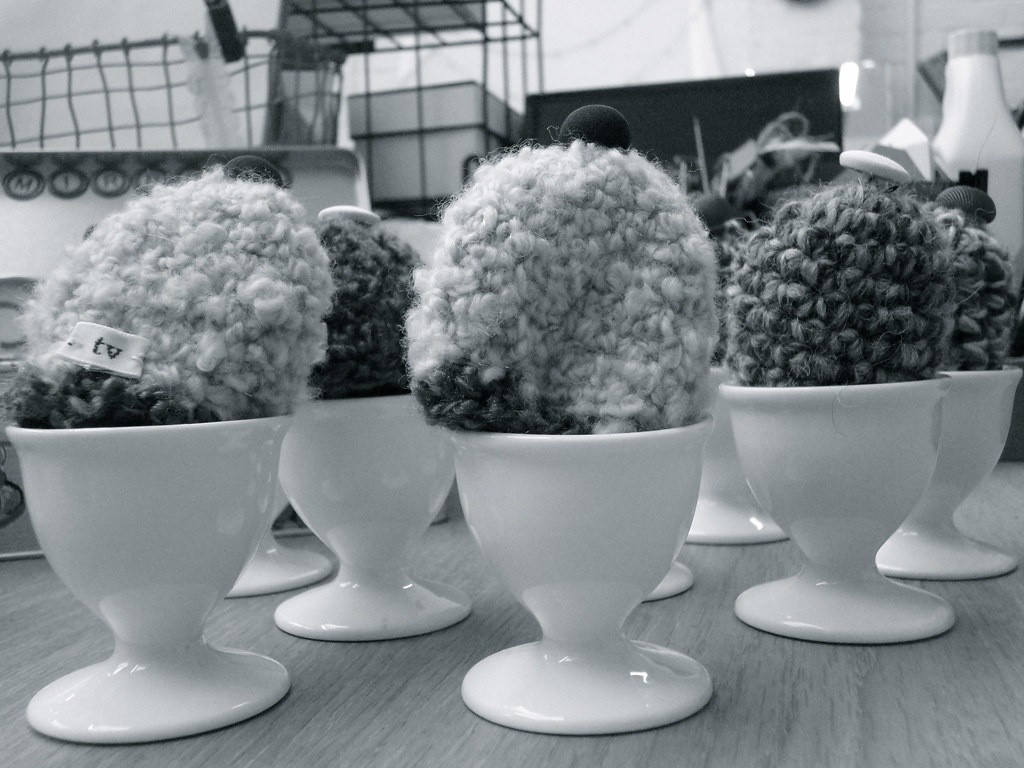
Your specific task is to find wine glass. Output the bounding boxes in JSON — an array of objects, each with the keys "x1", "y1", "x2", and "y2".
[
  {"x1": 687, "y1": 366, "x2": 788, "y2": 546},
  {"x1": 278, "y1": 393, "x2": 473, "y2": 642},
  {"x1": 718, "y1": 375, "x2": 957, "y2": 645},
  {"x1": 226, "y1": 403, "x2": 334, "y2": 599},
  {"x1": 4, "y1": 414, "x2": 297, "y2": 747},
  {"x1": 874, "y1": 366, "x2": 1024, "y2": 580},
  {"x1": 449, "y1": 411, "x2": 717, "y2": 736}
]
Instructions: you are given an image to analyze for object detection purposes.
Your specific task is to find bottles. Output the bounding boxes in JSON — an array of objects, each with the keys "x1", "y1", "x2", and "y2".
[{"x1": 929, "y1": 28, "x2": 1024, "y2": 284}]
